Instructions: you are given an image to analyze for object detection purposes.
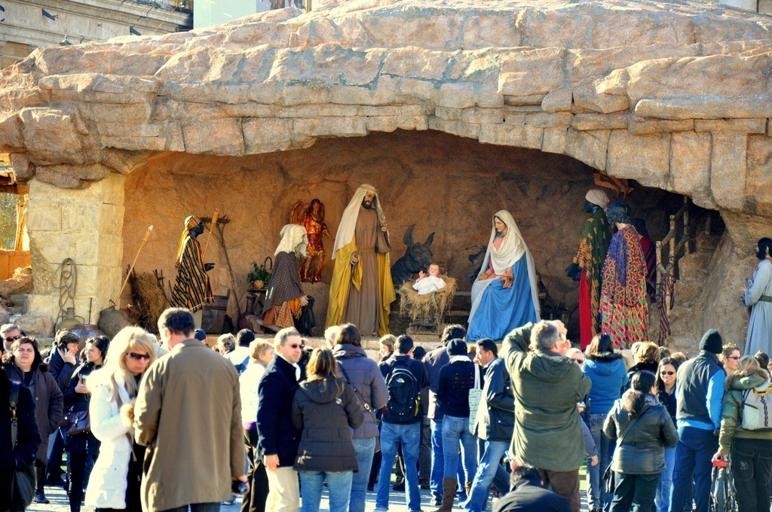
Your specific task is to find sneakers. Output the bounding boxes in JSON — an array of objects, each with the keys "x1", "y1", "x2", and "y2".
[
  {"x1": 420, "y1": 479, "x2": 430, "y2": 490},
  {"x1": 45, "y1": 475, "x2": 66, "y2": 487},
  {"x1": 392, "y1": 477, "x2": 406, "y2": 492},
  {"x1": 34, "y1": 492, "x2": 50, "y2": 503}
]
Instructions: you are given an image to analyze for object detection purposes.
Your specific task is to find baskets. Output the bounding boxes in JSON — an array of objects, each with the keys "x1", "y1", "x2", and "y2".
[{"x1": 249, "y1": 256, "x2": 273, "y2": 289}]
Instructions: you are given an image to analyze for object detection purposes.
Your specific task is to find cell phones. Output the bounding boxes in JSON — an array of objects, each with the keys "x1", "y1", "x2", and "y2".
[{"x1": 57, "y1": 342, "x2": 69, "y2": 354}]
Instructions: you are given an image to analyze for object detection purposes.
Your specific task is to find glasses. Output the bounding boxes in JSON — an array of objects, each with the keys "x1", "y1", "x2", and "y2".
[
  {"x1": 126, "y1": 351, "x2": 151, "y2": 361},
  {"x1": 4, "y1": 334, "x2": 21, "y2": 341}
]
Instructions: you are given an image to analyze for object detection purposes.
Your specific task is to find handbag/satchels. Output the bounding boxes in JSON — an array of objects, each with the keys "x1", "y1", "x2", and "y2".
[
  {"x1": 64, "y1": 409, "x2": 93, "y2": 436},
  {"x1": 467, "y1": 363, "x2": 486, "y2": 435},
  {"x1": 12, "y1": 453, "x2": 36, "y2": 508},
  {"x1": 602, "y1": 462, "x2": 616, "y2": 494}
]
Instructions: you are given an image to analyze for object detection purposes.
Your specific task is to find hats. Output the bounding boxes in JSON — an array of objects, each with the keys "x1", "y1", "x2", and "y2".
[
  {"x1": 699, "y1": 328, "x2": 723, "y2": 354},
  {"x1": 195, "y1": 329, "x2": 206, "y2": 341}
]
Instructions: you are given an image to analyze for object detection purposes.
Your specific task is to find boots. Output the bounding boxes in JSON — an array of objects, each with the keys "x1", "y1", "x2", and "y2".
[
  {"x1": 464, "y1": 481, "x2": 473, "y2": 498},
  {"x1": 431, "y1": 478, "x2": 457, "y2": 512}
]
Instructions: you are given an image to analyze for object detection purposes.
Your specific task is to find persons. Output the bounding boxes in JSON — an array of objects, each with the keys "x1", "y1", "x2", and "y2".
[
  {"x1": 416, "y1": 261, "x2": 448, "y2": 297},
  {"x1": 412, "y1": 266, "x2": 427, "y2": 290},
  {"x1": 599, "y1": 201, "x2": 649, "y2": 353},
  {"x1": 169, "y1": 213, "x2": 216, "y2": 316},
  {"x1": 613, "y1": 216, "x2": 657, "y2": 304},
  {"x1": 300, "y1": 196, "x2": 330, "y2": 284},
  {"x1": 738, "y1": 236, "x2": 772, "y2": 357},
  {"x1": 534, "y1": 270, "x2": 556, "y2": 321},
  {"x1": 256, "y1": 200, "x2": 309, "y2": 330},
  {"x1": 464, "y1": 208, "x2": 542, "y2": 345},
  {"x1": 324, "y1": 182, "x2": 398, "y2": 339},
  {"x1": 571, "y1": 188, "x2": 612, "y2": 353},
  {"x1": 0, "y1": 304, "x2": 772, "y2": 512}
]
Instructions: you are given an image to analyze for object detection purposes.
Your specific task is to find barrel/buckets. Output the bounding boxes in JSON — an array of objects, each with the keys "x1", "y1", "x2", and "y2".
[{"x1": 202, "y1": 289, "x2": 230, "y2": 333}]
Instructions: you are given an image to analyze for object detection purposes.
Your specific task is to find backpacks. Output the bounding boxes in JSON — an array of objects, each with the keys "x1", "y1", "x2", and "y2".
[
  {"x1": 739, "y1": 376, "x2": 772, "y2": 432},
  {"x1": 380, "y1": 360, "x2": 422, "y2": 420}
]
